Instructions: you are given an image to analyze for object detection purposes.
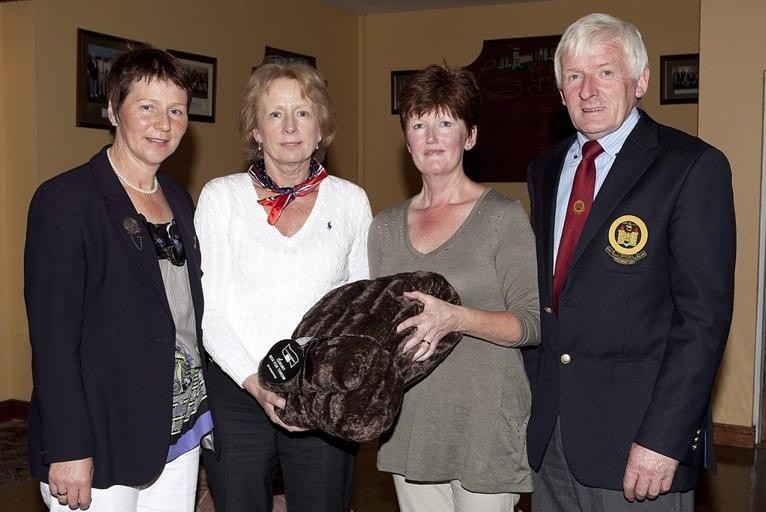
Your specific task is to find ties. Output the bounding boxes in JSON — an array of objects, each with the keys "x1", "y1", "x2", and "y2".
[{"x1": 552, "y1": 141, "x2": 605, "y2": 314}]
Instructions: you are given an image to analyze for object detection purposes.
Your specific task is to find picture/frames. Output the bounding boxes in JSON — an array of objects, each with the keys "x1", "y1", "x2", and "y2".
[
  {"x1": 167, "y1": 49, "x2": 217, "y2": 122},
  {"x1": 660, "y1": 54, "x2": 699, "y2": 104},
  {"x1": 76, "y1": 28, "x2": 151, "y2": 129},
  {"x1": 391, "y1": 70, "x2": 420, "y2": 114}
]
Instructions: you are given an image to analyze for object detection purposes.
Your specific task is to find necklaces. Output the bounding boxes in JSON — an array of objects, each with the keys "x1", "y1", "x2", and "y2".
[{"x1": 106, "y1": 147, "x2": 160, "y2": 195}]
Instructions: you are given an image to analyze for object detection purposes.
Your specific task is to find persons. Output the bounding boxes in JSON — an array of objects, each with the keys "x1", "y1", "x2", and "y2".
[
  {"x1": 24, "y1": 49, "x2": 224, "y2": 512},
  {"x1": 190, "y1": 59, "x2": 372, "y2": 512},
  {"x1": 519, "y1": 11, "x2": 738, "y2": 511},
  {"x1": 366, "y1": 62, "x2": 542, "y2": 511},
  {"x1": 673, "y1": 65, "x2": 700, "y2": 90},
  {"x1": 106, "y1": 58, "x2": 114, "y2": 75},
  {"x1": 97, "y1": 56, "x2": 106, "y2": 98},
  {"x1": 87, "y1": 50, "x2": 98, "y2": 97}
]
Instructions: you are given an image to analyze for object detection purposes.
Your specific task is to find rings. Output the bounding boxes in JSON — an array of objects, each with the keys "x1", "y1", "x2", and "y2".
[
  {"x1": 422, "y1": 339, "x2": 431, "y2": 345},
  {"x1": 57, "y1": 491, "x2": 67, "y2": 496}
]
